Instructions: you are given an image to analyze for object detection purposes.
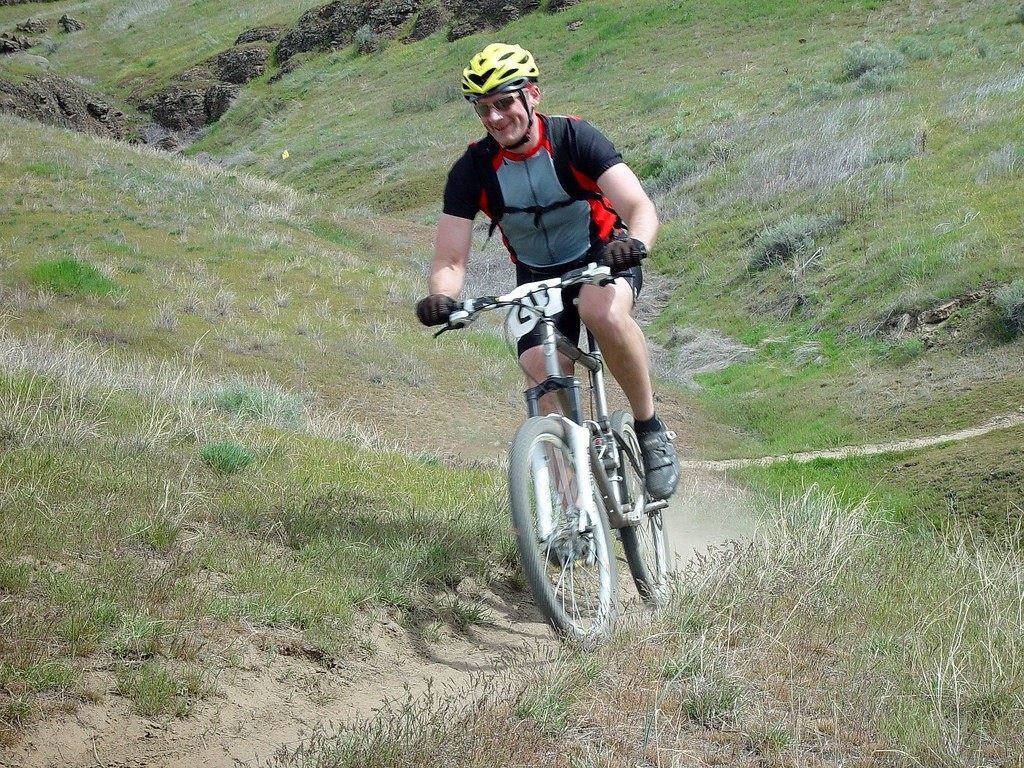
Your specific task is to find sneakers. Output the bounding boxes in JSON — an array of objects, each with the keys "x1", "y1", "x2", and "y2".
[
  {"x1": 637, "y1": 414, "x2": 680, "y2": 498},
  {"x1": 550, "y1": 535, "x2": 588, "y2": 572}
]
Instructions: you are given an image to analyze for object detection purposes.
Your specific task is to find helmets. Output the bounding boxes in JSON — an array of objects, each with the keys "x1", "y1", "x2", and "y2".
[{"x1": 461, "y1": 43, "x2": 540, "y2": 103}]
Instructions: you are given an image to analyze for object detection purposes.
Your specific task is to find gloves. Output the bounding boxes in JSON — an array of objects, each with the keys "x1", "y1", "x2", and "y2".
[
  {"x1": 416, "y1": 294, "x2": 456, "y2": 327},
  {"x1": 600, "y1": 238, "x2": 647, "y2": 269}
]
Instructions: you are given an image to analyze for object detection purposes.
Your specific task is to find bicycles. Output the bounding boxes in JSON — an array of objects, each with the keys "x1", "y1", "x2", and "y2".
[{"x1": 432, "y1": 250, "x2": 670, "y2": 652}]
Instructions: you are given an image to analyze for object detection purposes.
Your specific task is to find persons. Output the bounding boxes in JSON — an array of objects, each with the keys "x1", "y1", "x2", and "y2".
[{"x1": 417, "y1": 42, "x2": 682, "y2": 566}]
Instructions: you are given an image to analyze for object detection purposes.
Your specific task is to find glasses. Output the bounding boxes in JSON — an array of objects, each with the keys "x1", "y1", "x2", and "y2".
[{"x1": 474, "y1": 88, "x2": 529, "y2": 117}]
